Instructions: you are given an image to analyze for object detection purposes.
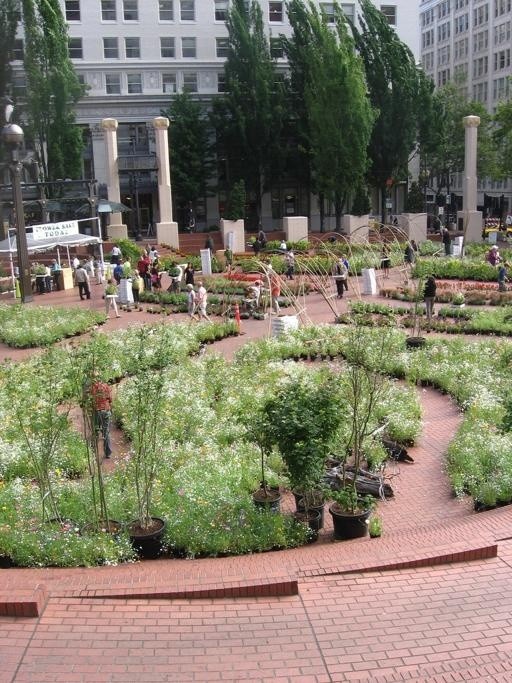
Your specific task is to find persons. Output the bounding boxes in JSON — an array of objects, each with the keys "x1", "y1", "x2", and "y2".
[
  {"x1": 484, "y1": 213, "x2": 512, "y2": 291},
  {"x1": 91, "y1": 377, "x2": 112, "y2": 459},
  {"x1": 81, "y1": 369, "x2": 92, "y2": 421},
  {"x1": 433, "y1": 214, "x2": 442, "y2": 233},
  {"x1": 442, "y1": 227, "x2": 451, "y2": 255},
  {"x1": 423, "y1": 277, "x2": 437, "y2": 319}
]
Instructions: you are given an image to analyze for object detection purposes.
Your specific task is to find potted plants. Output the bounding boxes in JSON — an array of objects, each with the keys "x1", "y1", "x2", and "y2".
[{"x1": 0, "y1": 181, "x2": 512, "y2": 569}]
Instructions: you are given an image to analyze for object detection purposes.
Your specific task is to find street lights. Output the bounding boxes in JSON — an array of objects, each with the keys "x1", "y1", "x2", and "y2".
[
  {"x1": 3, "y1": 123, "x2": 39, "y2": 302},
  {"x1": 422, "y1": 149, "x2": 430, "y2": 212}
]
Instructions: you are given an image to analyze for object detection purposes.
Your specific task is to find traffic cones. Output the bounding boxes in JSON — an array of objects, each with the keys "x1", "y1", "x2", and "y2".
[
  {"x1": 14, "y1": 279, "x2": 23, "y2": 300},
  {"x1": 103, "y1": 265, "x2": 112, "y2": 281}
]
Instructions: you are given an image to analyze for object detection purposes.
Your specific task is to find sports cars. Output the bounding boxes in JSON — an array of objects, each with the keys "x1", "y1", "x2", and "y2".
[{"x1": 484, "y1": 223, "x2": 512, "y2": 238}]
